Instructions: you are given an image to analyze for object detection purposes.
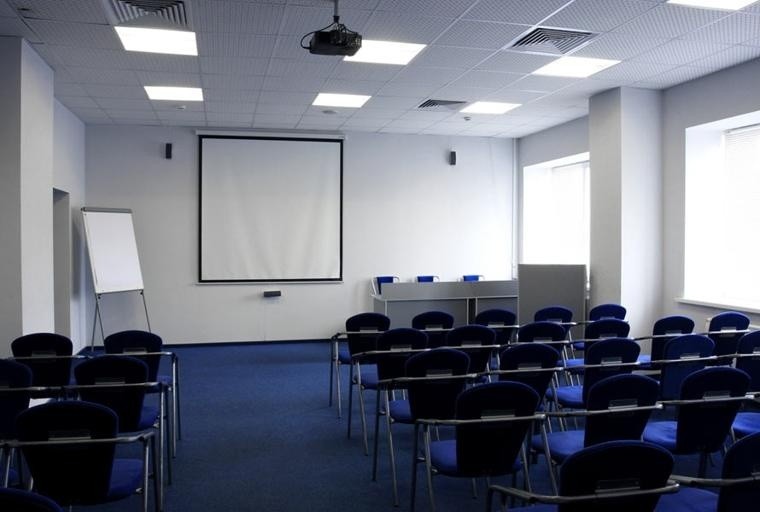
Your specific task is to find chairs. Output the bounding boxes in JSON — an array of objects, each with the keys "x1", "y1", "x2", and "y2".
[
  {"x1": 415, "y1": 274, "x2": 439, "y2": 283},
  {"x1": 332, "y1": 304, "x2": 760, "y2": 510},
  {"x1": 0, "y1": 329, "x2": 179, "y2": 509},
  {"x1": 457, "y1": 274, "x2": 483, "y2": 281},
  {"x1": 372, "y1": 275, "x2": 400, "y2": 296}
]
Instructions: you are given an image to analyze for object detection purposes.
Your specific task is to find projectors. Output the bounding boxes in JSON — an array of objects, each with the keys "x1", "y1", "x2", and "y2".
[{"x1": 308, "y1": 30, "x2": 362, "y2": 56}]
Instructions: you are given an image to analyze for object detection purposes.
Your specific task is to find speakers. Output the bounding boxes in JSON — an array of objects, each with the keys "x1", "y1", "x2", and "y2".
[
  {"x1": 450, "y1": 151, "x2": 456, "y2": 165},
  {"x1": 166, "y1": 143, "x2": 173, "y2": 158}
]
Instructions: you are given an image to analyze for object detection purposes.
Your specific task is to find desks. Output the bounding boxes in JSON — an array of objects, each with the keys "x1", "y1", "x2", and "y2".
[{"x1": 364, "y1": 276, "x2": 518, "y2": 333}]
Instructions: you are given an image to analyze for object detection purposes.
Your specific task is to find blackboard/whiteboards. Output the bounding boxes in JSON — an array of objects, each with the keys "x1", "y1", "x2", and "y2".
[{"x1": 81, "y1": 207, "x2": 145, "y2": 294}]
